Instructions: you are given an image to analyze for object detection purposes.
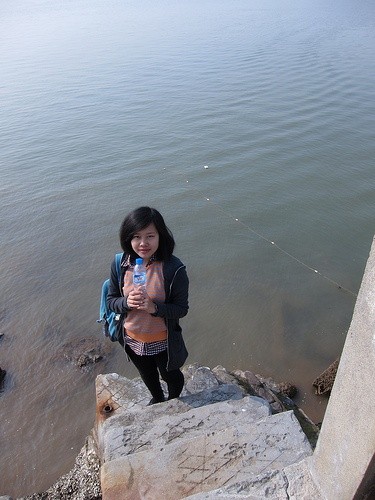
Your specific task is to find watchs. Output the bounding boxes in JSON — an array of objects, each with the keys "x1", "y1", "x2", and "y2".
[{"x1": 150, "y1": 304, "x2": 160, "y2": 317}]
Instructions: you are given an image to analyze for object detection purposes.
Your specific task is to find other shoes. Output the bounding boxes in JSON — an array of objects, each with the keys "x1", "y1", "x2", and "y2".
[{"x1": 146, "y1": 397, "x2": 165, "y2": 406}]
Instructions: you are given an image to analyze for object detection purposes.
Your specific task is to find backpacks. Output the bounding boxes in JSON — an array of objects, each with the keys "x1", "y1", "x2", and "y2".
[{"x1": 98, "y1": 252, "x2": 129, "y2": 343}]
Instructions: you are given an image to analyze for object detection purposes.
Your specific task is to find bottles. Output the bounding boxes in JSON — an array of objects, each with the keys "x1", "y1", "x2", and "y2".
[{"x1": 133, "y1": 258, "x2": 147, "y2": 297}]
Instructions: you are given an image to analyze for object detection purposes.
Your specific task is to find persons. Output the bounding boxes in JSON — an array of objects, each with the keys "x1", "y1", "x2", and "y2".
[{"x1": 105, "y1": 206, "x2": 189, "y2": 408}]
